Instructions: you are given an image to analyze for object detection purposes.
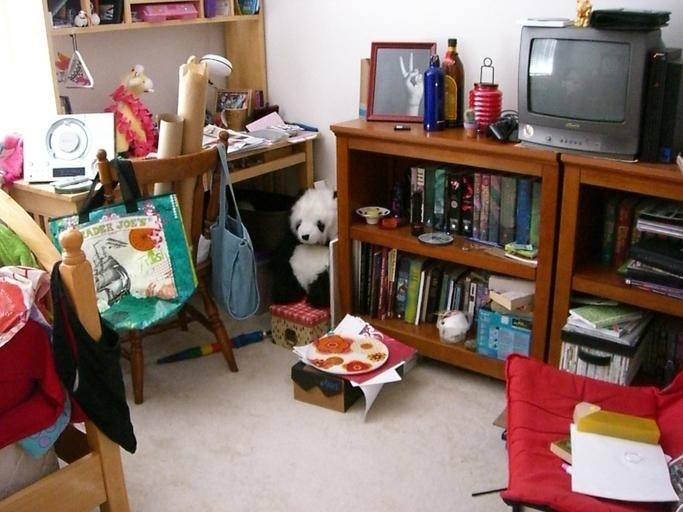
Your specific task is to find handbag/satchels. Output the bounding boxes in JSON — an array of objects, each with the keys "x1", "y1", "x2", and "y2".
[
  {"x1": 48, "y1": 158, "x2": 199, "y2": 335},
  {"x1": 48, "y1": 257, "x2": 138, "y2": 454},
  {"x1": 210, "y1": 144, "x2": 260, "y2": 320}
]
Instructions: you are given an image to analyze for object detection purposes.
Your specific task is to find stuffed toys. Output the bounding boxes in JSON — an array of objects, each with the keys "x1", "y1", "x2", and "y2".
[
  {"x1": 113, "y1": 65, "x2": 156, "y2": 156},
  {"x1": 270, "y1": 181, "x2": 337, "y2": 310}
]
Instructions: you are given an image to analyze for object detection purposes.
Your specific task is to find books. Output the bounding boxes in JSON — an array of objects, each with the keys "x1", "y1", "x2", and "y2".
[
  {"x1": 564, "y1": 192, "x2": 683, "y2": 375},
  {"x1": 352, "y1": 237, "x2": 533, "y2": 326},
  {"x1": 409, "y1": 167, "x2": 542, "y2": 251},
  {"x1": 549, "y1": 401, "x2": 683, "y2": 512},
  {"x1": 200, "y1": 110, "x2": 315, "y2": 152}
]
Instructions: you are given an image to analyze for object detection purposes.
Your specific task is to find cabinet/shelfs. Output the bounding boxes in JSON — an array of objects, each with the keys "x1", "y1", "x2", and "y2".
[
  {"x1": 41, "y1": 0, "x2": 266, "y2": 39},
  {"x1": 320, "y1": 119, "x2": 558, "y2": 393}
]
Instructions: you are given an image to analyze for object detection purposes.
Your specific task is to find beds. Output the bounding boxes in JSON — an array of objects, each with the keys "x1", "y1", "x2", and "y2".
[{"x1": 0, "y1": 188, "x2": 131, "y2": 511}]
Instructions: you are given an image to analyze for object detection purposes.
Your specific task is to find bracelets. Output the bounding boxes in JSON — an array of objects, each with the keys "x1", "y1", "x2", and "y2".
[{"x1": 408, "y1": 102, "x2": 419, "y2": 107}]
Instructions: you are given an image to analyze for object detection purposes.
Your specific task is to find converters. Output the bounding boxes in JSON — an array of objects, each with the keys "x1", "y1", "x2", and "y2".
[{"x1": 489, "y1": 116, "x2": 517, "y2": 141}]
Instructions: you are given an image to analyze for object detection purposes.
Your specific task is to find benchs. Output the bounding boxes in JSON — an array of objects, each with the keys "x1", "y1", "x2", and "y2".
[{"x1": 492, "y1": 351, "x2": 682, "y2": 512}]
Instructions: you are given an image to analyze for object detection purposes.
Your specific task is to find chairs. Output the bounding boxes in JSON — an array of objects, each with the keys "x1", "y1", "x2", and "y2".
[{"x1": 96, "y1": 130, "x2": 238, "y2": 405}]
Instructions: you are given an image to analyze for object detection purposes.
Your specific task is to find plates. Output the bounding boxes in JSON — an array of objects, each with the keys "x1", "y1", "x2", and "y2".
[
  {"x1": 305, "y1": 336, "x2": 390, "y2": 372},
  {"x1": 355, "y1": 206, "x2": 389, "y2": 225}
]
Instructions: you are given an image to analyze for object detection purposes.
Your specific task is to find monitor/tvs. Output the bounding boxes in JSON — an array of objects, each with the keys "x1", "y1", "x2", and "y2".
[{"x1": 517, "y1": 24, "x2": 665, "y2": 161}]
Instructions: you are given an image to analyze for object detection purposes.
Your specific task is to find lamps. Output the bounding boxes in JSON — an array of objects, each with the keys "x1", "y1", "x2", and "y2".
[{"x1": 199, "y1": 53, "x2": 233, "y2": 89}]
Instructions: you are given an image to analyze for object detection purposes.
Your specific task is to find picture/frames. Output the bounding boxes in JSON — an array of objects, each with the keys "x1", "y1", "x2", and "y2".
[
  {"x1": 366, "y1": 41, "x2": 436, "y2": 123},
  {"x1": 211, "y1": 88, "x2": 253, "y2": 118}
]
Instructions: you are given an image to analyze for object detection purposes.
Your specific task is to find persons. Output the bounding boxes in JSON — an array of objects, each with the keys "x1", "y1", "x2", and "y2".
[{"x1": 397, "y1": 52, "x2": 423, "y2": 116}]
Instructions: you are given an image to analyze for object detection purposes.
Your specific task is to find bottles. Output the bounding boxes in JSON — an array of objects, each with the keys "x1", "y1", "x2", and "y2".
[
  {"x1": 423, "y1": 57, "x2": 444, "y2": 129},
  {"x1": 442, "y1": 38, "x2": 463, "y2": 129}
]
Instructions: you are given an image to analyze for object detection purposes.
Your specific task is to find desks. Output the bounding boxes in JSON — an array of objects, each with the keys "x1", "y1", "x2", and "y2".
[
  {"x1": 12, "y1": 121, "x2": 317, "y2": 241},
  {"x1": 543, "y1": 152, "x2": 683, "y2": 385}
]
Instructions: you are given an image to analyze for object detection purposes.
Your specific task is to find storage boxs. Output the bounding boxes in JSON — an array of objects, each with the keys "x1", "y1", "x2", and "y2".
[
  {"x1": 288, "y1": 358, "x2": 364, "y2": 412},
  {"x1": 555, "y1": 321, "x2": 653, "y2": 388},
  {"x1": 472, "y1": 300, "x2": 531, "y2": 363}
]
